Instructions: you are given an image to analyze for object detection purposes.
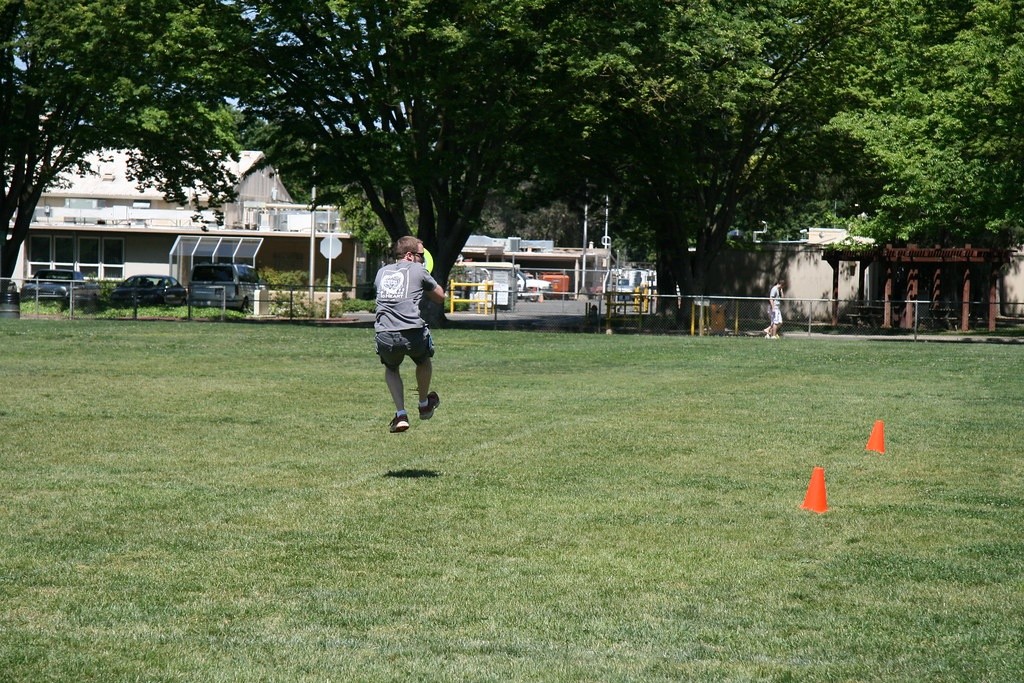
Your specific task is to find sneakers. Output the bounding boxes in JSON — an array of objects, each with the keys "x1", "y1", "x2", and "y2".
[
  {"x1": 418, "y1": 391, "x2": 439, "y2": 420},
  {"x1": 390, "y1": 413, "x2": 409, "y2": 433}
]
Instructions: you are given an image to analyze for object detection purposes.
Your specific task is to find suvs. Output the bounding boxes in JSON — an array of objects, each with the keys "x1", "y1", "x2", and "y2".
[{"x1": 517, "y1": 269, "x2": 553, "y2": 302}]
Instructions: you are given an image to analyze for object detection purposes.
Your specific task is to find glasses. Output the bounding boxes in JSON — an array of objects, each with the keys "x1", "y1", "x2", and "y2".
[{"x1": 405, "y1": 251, "x2": 424, "y2": 257}]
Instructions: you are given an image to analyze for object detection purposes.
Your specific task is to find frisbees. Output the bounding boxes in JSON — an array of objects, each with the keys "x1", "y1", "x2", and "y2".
[{"x1": 422, "y1": 247, "x2": 434, "y2": 274}]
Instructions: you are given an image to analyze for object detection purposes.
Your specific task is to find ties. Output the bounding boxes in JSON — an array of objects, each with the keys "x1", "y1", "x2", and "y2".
[{"x1": 779, "y1": 289, "x2": 781, "y2": 298}]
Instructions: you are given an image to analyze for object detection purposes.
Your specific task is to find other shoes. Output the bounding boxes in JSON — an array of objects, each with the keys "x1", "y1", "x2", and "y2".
[
  {"x1": 776, "y1": 334, "x2": 779, "y2": 338},
  {"x1": 765, "y1": 334, "x2": 770, "y2": 339},
  {"x1": 771, "y1": 336, "x2": 777, "y2": 339},
  {"x1": 764, "y1": 328, "x2": 770, "y2": 333}
]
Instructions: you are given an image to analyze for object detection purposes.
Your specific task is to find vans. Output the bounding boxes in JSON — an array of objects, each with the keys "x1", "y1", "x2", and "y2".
[{"x1": 187, "y1": 263, "x2": 267, "y2": 313}]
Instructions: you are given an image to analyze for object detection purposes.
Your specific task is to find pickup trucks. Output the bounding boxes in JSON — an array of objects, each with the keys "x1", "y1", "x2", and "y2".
[{"x1": 21, "y1": 269, "x2": 101, "y2": 314}]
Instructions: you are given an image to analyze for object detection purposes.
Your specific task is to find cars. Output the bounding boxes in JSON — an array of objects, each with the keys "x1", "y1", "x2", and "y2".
[{"x1": 110, "y1": 274, "x2": 187, "y2": 308}]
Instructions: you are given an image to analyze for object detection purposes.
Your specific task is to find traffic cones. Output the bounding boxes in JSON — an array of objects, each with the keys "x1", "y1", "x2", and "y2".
[
  {"x1": 865, "y1": 419, "x2": 885, "y2": 454},
  {"x1": 799, "y1": 466, "x2": 830, "y2": 513}
]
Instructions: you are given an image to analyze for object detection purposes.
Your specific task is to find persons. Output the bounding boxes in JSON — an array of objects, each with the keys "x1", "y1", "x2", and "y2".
[
  {"x1": 763, "y1": 279, "x2": 785, "y2": 339},
  {"x1": 371, "y1": 236, "x2": 446, "y2": 432}
]
